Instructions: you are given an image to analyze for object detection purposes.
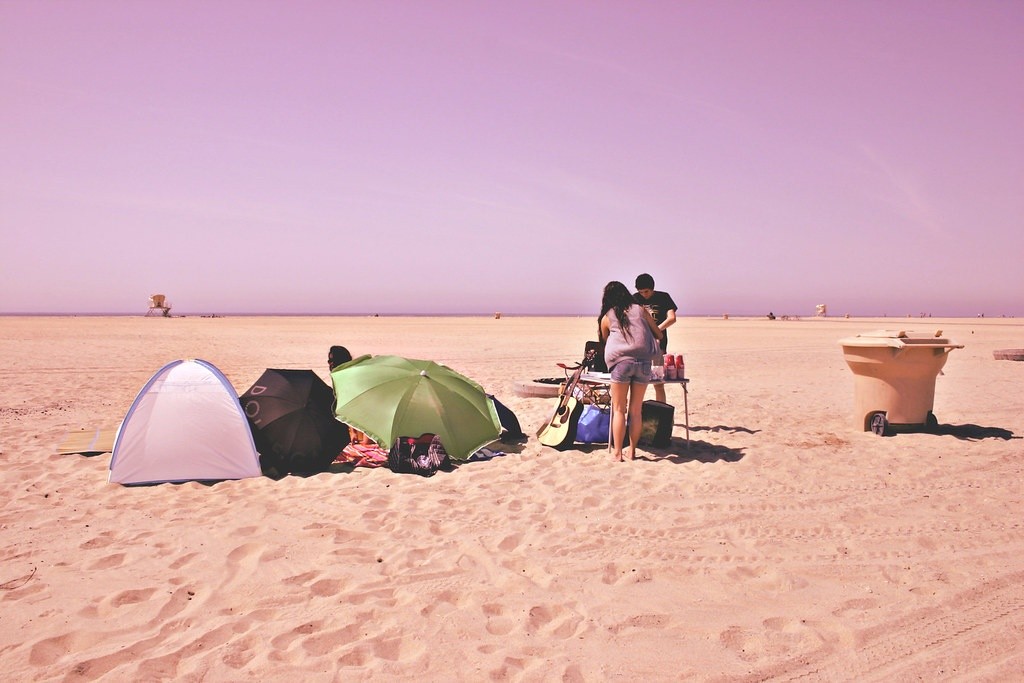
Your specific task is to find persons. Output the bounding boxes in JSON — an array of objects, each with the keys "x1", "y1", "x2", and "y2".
[
  {"x1": 327, "y1": 345, "x2": 352, "y2": 372},
  {"x1": 631, "y1": 274, "x2": 678, "y2": 404},
  {"x1": 599, "y1": 282, "x2": 663, "y2": 463}
]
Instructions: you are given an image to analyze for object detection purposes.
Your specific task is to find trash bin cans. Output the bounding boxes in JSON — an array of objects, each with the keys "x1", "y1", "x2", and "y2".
[{"x1": 838, "y1": 328, "x2": 965, "y2": 437}]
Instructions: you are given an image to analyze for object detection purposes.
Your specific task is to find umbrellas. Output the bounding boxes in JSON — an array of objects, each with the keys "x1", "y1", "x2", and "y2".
[
  {"x1": 238, "y1": 368, "x2": 351, "y2": 478},
  {"x1": 330, "y1": 353, "x2": 507, "y2": 461}
]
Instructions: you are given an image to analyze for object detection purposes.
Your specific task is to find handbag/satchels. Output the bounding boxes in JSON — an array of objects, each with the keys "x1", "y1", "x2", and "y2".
[{"x1": 385, "y1": 433, "x2": 451, "y2": 477}]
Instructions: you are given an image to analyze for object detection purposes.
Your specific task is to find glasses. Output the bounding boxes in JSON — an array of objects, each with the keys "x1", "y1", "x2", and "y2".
[{"x1": 327, "y1": 358, "x2": 342, "y2": 365}]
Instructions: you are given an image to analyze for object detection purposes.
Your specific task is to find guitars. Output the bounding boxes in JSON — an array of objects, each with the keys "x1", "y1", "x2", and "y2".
[{"x1": 536, "y1": 348, "x2": 598, "y2": 452}]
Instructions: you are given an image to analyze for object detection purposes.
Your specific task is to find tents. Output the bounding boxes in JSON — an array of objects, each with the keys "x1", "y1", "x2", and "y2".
[{"x1": 108, "y1": 359, "x2": 262, "y2": 487}]
